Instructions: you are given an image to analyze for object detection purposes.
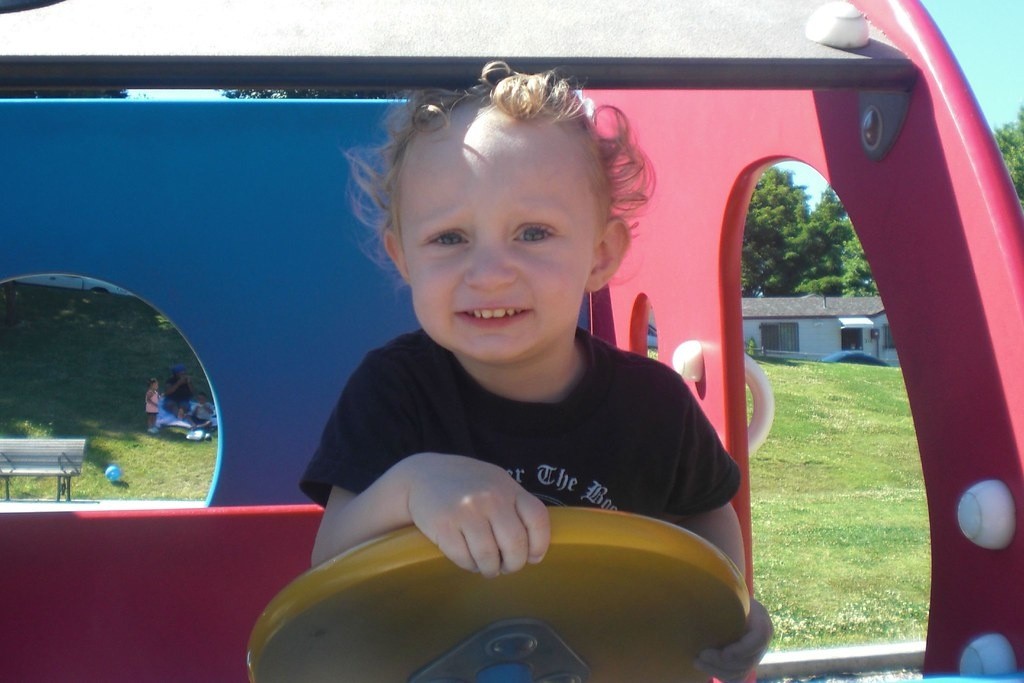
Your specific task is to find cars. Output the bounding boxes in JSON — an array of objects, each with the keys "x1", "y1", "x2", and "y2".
[
  {"x1": 15, "y1": 275, "x2": 133, "y2": 296},
  {"x1": 647, "y1": 324, "x2": 658, "y2": 348},
  {"x1": 820, "y1": 350, "x2": 892, "y2": 367}
]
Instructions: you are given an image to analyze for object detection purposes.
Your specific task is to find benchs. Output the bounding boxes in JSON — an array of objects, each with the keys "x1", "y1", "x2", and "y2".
[
  {"x1": 0, "y1": 438, "x2": 87, "y2": 503},
  {"x1": 0, "y1": 504, "x2": 327, "y2": 683}
]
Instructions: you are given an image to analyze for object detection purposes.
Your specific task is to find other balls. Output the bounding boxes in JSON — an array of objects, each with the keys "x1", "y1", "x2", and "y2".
[{"x1": 105, "y1": 466, "x2": 120, "y2": 481}]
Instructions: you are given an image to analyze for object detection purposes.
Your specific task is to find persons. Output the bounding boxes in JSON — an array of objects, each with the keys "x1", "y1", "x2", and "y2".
[
  {"x1": 144, "y1": 377, "x2": 161, "y2": 434},
  {"x1": 185, "y1": 392, "x2": 216, "y2": 431},
  {"x1": 299, "y1": 60, "x2": 776, "y2": 682},
  {"x1": 161, "y1": 365, "x2": 196, "y2": 421}
]
